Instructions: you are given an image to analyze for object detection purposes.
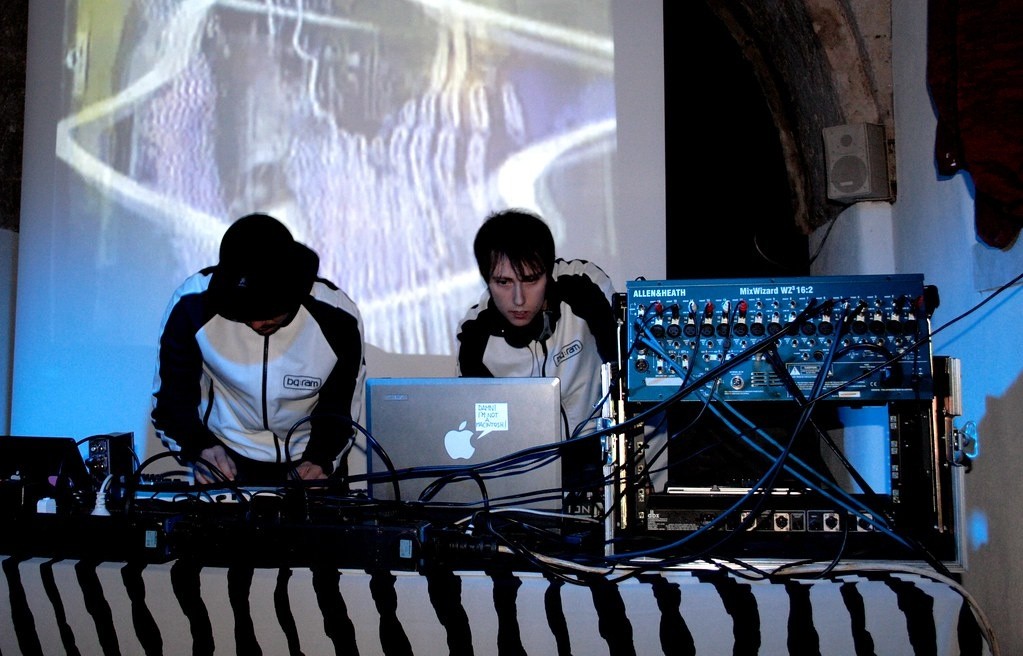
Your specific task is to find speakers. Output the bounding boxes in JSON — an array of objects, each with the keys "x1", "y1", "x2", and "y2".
[{"x1": 822, "y1": 122, "x2": 891, "y2": 202}]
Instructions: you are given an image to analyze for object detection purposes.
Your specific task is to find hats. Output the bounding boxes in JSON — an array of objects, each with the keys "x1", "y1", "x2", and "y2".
[{"x1": 207, "y1": 214, "x2": 319, "y2": 323}]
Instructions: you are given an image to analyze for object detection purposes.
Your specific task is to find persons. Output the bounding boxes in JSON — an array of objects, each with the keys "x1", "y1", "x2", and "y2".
[
  {"x1": 150, "y1": 213, "x2": 367, "y2": 495},
  {"x1": 456, "y1": 209, "x2": 617, "y2": 490}
]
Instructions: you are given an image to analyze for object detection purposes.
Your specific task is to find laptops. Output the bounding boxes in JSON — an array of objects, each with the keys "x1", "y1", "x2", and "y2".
[
  {"x1": 0, "y1": 435, "x2": 93, "y2": 503},
  {"x1": 364, "y1": 375, "x2": 564, "y2": 510}
]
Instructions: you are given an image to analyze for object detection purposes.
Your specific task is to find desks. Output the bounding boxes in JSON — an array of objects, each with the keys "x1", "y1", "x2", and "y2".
[{"x1": 0, "y1": 556, "x2": 999, "y2": 656}]
